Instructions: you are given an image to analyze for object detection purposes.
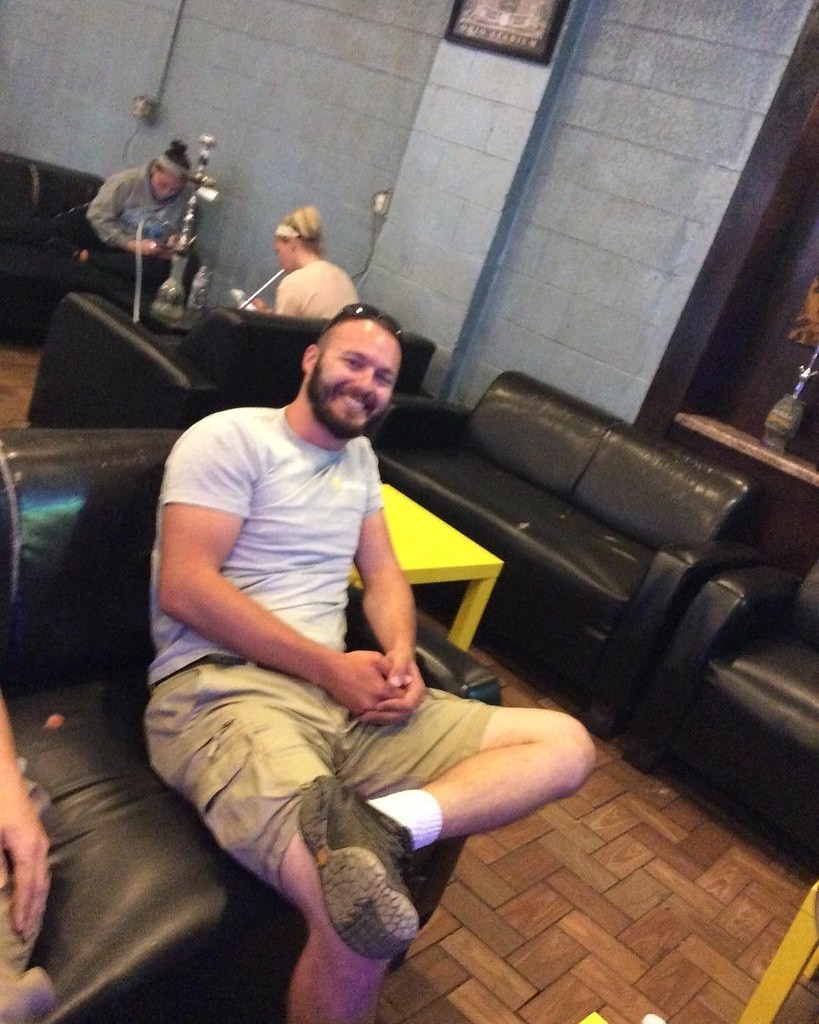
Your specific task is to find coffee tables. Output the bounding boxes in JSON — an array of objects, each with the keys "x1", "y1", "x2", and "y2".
[{"x1": 348, "y1": 481, "x2": 502, "y2": 654}]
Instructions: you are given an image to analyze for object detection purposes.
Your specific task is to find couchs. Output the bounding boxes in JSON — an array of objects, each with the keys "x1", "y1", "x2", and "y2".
[
  {"x1": 622, "y1": 550, "x2": 819, "y2": 881},
  {"x1": 26, "y1": 291, "x2": 437, "y2": 427},
  {"x1": 366, "y1": 371, "x2": 766, "y2": 740},
  {"x1": 0, "y1": 425, "x2": 503, "y2": 1024},
  {"x1": 0, "y1": 149, "x2": 202, "y2": 347}
]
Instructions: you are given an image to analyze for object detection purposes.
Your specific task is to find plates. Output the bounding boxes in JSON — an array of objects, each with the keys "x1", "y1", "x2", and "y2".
[{"x1": 181, "y1": 168, "x2": 217, "y2": 186}]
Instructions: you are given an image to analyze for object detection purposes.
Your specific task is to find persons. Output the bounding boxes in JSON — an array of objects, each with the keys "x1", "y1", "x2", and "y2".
[
  {"x1": 0, "y1": 692, "x2": 55, "y2": 1024},
  {"x1": 141, "y1": 306, "x2": 595, "y2": 1024},
  {"x1": 71, "y1": 139, "x2": 202, "y2": 293},
  {"x1": 242, "y1": 207, "x2": 360, "y2": 323}
]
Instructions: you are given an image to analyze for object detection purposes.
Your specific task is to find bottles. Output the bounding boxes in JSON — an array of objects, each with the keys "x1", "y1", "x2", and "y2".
[{"x1": 186, "y1": 265, "x2": 210, "y2": 310}]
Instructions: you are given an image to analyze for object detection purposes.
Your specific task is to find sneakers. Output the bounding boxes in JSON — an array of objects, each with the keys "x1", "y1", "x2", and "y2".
[{"x1": 296, "y1": 774, "x2": 430, "y2": 960}]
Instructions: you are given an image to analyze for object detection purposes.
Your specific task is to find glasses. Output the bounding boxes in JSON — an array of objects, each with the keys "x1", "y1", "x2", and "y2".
[{"x1": 320, "y1": 302, "x2": 407, "y2": 344}]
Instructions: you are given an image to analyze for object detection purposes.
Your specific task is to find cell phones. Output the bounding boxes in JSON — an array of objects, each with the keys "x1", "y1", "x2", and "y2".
[
  {"x1": 230, "y1": 288, "x2": 257, "y2": 312},
  {"x1": 150, "y1": 243, "x2": 170, "y2": 251}
]
